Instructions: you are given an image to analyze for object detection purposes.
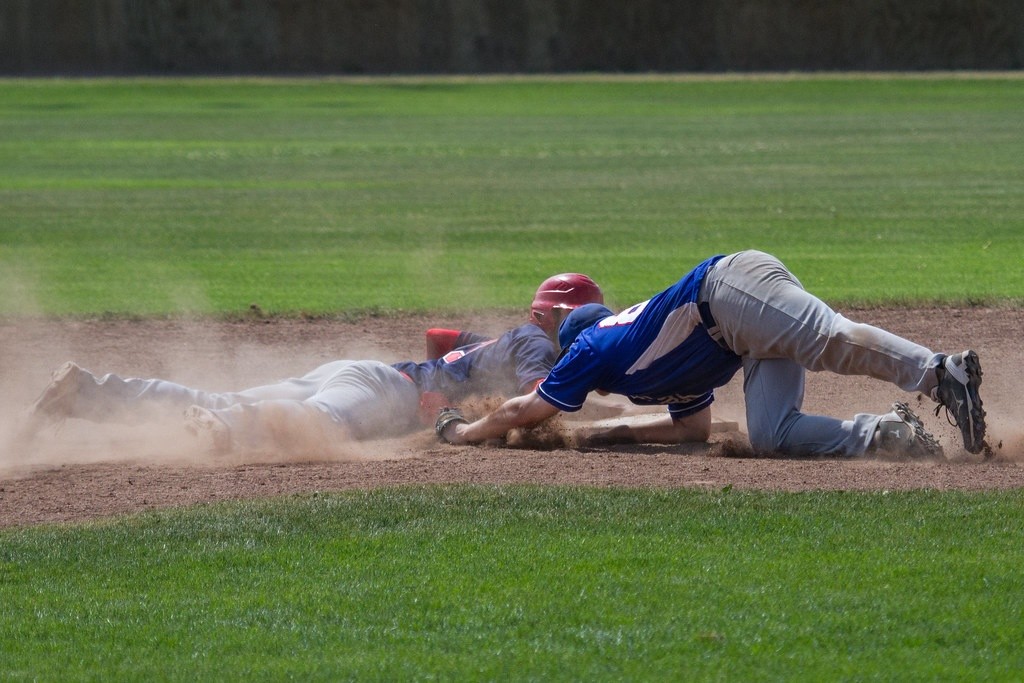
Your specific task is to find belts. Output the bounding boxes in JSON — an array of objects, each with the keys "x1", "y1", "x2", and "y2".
[{"x1": 698, "y1": 302, "x2": 732, "y2": 354}]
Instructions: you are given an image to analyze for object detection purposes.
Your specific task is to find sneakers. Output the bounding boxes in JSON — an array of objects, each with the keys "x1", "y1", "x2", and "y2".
[
  {"x1": 183, "y1": 405, "x2": 239, "y2": 462},
  {"x1": 931, "y1": 350, "x2": 986, "y2": 455},
  {"x1": 878, "y1": 402, "x2": 948, "y2": 463},
  {"x1": 12, "y1": 361, "x2": 79, "y2": 442}
]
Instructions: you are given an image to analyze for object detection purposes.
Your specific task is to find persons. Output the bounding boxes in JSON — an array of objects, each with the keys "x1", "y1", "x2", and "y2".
[
  {"x1": 17, "y1": 273, "x2": 673, "y2": 473},
  {"x1": 434, "y1": 248, "x2": 986, "y2": 459}
]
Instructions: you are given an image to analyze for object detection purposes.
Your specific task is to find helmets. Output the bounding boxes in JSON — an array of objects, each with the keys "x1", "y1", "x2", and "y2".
[{"x1": 528, "y1": 273, "x2": 603, "y2": 339}]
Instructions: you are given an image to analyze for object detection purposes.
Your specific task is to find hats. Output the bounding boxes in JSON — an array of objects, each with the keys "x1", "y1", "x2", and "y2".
[{"x1": 554, "y1": 302, "x2": 614, "y2": 364}]
